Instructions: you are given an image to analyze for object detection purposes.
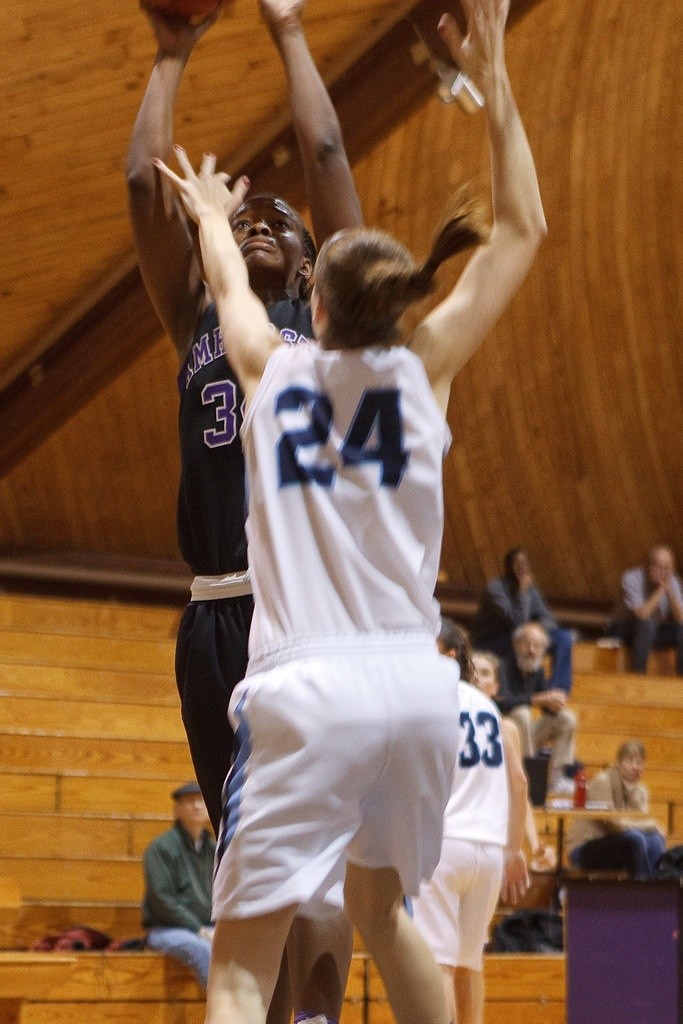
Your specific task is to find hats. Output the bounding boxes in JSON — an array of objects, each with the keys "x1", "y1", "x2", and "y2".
[{"x1": 171, "y1": 782, "x2": 200, "y2": 797}]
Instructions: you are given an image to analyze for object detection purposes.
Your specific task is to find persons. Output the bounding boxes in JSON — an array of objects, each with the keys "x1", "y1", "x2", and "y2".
[
  {"x1": 400, "y1": 616, "x2": 532, "y2": 1024},
  {"x1": 467, "y1": 651, "x2": 550, "y2": 863},
  {"x1": 120, "y1": 0, "x2": 370, "y2": 1024},
  {"x1": 565, "y1": 742, "x2": 669, "y2": 882},
  {"x1": 493, "y1": 623, "x2": 576, "y2": 800},
  {"x1": 143, "y1": 785, "x2": 217, "y2": 987},
  {"x1": 151, "y1": 0, "x2": 550, "y2": 1024},
  {"x1": 612, "y1": 545, "x2": 683, "y2": 675},
  {"x1": 490, "y1": 912, "x2": 565, "y2": 953},
  {"x1": 468, "y1": 549, "x2": 571, "y2": 694}
]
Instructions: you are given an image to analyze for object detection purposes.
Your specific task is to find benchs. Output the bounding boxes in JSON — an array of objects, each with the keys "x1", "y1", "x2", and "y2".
[{"x1": 0, "y1": 591, "x2": 683, "y2": 1024}]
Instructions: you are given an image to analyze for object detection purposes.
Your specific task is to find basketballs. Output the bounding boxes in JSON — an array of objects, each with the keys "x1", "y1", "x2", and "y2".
[{"x1": 142, "y1": 1, "x2": 222, "y2": 15}]
